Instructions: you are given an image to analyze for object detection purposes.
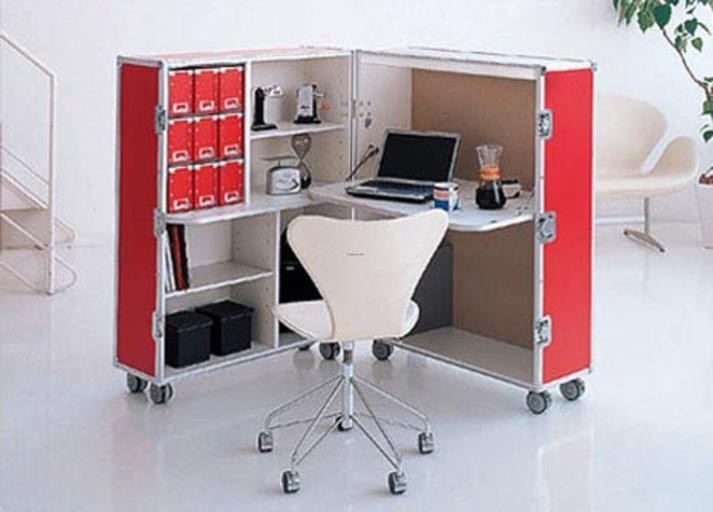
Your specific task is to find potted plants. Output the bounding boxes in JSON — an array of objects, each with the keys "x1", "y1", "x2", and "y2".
[{"x1": 608, "y1": 0, "x2": 713, "y2": 250}]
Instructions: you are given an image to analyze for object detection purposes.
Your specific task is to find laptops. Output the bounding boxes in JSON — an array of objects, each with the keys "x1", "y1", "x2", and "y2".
[{"x1": 346, "y1": 127, "x2": 462, "y2": 204}]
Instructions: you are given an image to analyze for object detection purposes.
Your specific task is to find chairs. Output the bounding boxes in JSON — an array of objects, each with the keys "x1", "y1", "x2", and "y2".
[
  {"x1": 257, "y1": 208, "x2": 449, "y2": 494},
  {"x1": 594, "y1": 89, "x2": 700, "y2": 254}
]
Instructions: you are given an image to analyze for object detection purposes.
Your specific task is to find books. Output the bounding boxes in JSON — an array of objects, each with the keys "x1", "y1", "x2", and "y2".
[{"x1": 164, "y1": 224, "x2": 189, "y2": 294}]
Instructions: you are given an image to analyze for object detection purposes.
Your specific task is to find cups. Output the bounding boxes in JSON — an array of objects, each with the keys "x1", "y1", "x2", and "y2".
[
  {"x1": 474, "y1": 144, "x2": 506, "y2": 210},
  {"x1": 432, "y1": 182, "x2": 461, "y2": 214}
]
